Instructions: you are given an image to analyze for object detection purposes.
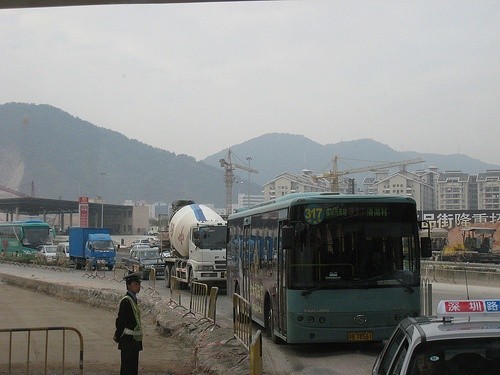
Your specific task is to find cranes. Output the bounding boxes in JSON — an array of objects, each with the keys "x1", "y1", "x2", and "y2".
[
  {"x1": 310, "y1": 154, "x2": 430, "y2": 193},
  {"x1": 218, "y1": 148, "x2": 262, "y2": 215}
]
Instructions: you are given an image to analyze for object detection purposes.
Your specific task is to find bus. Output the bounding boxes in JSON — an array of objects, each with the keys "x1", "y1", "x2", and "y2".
[
  {"x1": 0, "y1": 221, "x2": 56, "y2": 260},
  {"x1": 225, "y1": 191, "x2": 433, "y2": 347}
]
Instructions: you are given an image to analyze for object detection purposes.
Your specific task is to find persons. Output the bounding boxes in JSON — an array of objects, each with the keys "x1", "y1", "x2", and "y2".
[{"x1": 113, "y1": 273, "x2": 143, "y2": 375}]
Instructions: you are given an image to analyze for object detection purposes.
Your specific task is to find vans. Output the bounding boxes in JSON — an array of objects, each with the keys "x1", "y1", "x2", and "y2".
[{"x1": 56, "y1": 242, "x2": 75, "y2": 267}]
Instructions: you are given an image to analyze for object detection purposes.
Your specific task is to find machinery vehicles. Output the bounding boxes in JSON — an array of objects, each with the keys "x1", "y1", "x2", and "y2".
[{"x1": 154, "y1": 199, "x2": 230, "y2": 292}]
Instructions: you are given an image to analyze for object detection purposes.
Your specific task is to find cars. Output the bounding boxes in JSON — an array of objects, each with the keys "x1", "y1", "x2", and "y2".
[
  {"x1": 372, "y1": 300, "x2": 500, "y2": 375},
  {"x1": 37, "y1": 244, "x2": 60, "y2": 265},
  {"x1": 120, "y1": 238, "x2": 170, "y2": 281}
]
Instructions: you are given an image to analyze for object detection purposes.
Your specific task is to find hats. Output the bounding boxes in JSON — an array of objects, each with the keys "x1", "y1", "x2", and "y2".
[{"x1": 123, "y1": 272, "x2": 143, "y2": 282}]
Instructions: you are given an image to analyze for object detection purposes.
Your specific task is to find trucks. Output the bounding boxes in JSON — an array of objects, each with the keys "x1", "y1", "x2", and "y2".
[{"x1": 67, "y1": 227, "x2": 119, "y2": 270}]
